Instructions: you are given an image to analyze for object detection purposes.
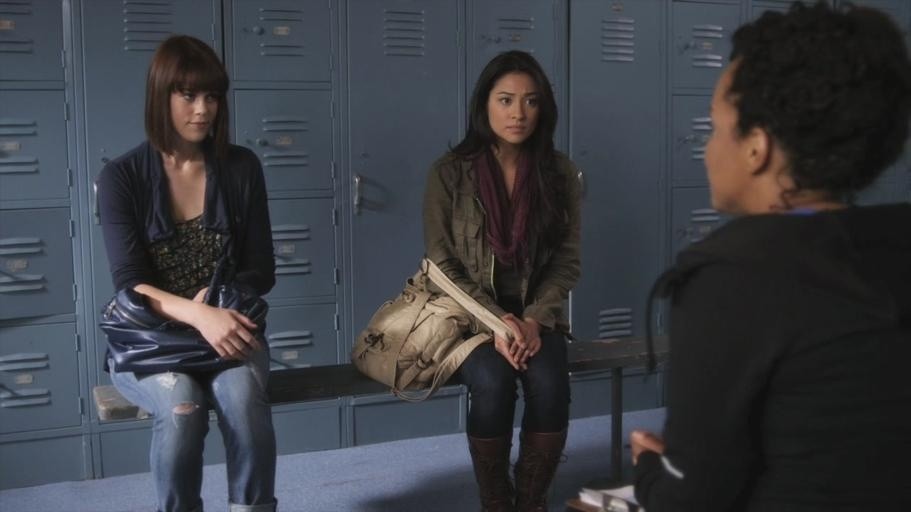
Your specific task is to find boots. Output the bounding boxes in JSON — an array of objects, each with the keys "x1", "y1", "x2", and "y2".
[
  {"x1": 461, "y1": 421, "x2": 514, "y2": 512},
  {"x1": 511, "y1": 426, "x2": 570, "y2": 512}
]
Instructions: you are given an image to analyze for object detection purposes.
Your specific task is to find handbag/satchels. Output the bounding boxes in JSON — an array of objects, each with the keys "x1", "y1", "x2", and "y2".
[
  {"x1": 349, "y1": 253, "x2": 515, "y2": 405},
  {"x1": 99, "y1": 285, "x2": 269, "y2": 380}
]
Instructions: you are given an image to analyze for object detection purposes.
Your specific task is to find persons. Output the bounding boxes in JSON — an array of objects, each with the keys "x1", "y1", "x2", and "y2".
[
  {"x1": 629, "y1": 0, "x2": 910, "y2": 512},
  {"x1": 423, "y1": 49, "x2": 585, "y2": 511},
  {"x1": 95, "y1": 36, "x2": 277, "y2": 512}
]
